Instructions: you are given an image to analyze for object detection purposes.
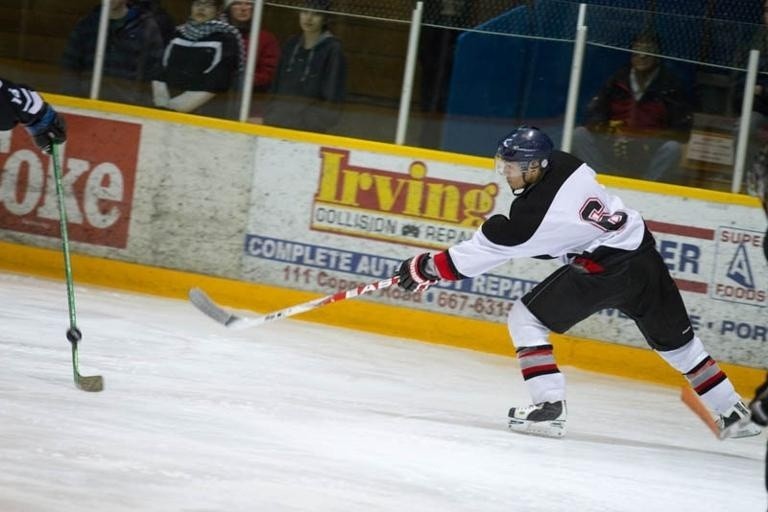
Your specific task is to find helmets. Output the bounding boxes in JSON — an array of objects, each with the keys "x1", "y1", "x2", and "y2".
[{"x1": 494, "y1": 123, "x2": 553, "y2": 171}]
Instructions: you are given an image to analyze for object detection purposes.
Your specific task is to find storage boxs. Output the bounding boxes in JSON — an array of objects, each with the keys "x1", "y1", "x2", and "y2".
[{"x1": 677, "y1": 129, "x2": 738, "y2": 175}]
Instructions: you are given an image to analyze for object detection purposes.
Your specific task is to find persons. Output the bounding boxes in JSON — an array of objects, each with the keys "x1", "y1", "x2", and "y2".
[
  {"x1": 394, "y1": 124, "x2": 753, "y2": 437},
  {"x1": 418, "y1": 0, "x2": 473, "y2": 149},
  {"x1": 0, "y1": 79, "x2": 66, "y2": 154},
  {"x1": 59, "y1": 1, "x2": 347, "y2": 134},
  {"x1": 733, "y1": 1, "x2": 767, "y2": 182},
  {"x1": 572, "y1": 34, "x2": 695, "y2": 183}
]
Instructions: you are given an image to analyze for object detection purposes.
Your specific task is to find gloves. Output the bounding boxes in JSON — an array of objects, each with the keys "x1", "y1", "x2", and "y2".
[
  {"x1": 399, "y1": 252, "x2": 442, "y2": 297},
  {"x1": 18, "y1": 99, "x2": 70, "y2": 156}
]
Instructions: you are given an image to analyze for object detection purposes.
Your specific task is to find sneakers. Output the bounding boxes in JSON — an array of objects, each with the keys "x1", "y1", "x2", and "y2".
[
  {"x1": 506, "y1": 399, "x2": 569, "y2": 424},
  {"x1": 712, "y1": 398, "x2": 753, "y2": 433}
]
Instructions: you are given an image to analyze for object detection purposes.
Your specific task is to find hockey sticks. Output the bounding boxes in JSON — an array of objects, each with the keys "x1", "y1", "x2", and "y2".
[
  {"x1": 187, "y1": 276, "x2": 399, "y2": 328},
  {"x1": 51, "y1": 142, "x2": 103, "y2": 391}
]
